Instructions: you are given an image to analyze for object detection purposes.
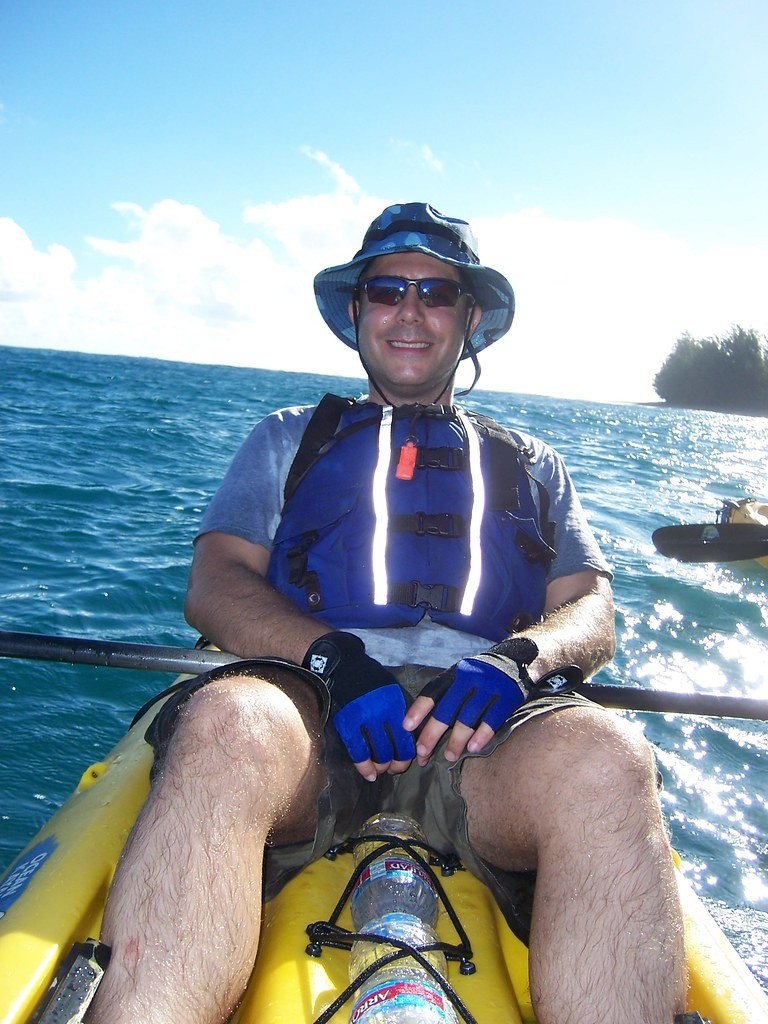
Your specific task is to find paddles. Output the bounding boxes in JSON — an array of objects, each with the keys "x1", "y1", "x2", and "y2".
[
  {"x1": 650, "y1": 523, "x2": 768, "y2": 563},
  {"x1": 0, "y1": 629, "x2": 768, "y2": 724}
]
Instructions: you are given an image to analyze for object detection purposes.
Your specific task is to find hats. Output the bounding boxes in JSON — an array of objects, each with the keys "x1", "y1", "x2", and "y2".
[{"x1": 313, "y1": 201, "x2": 515, "y2": 360}]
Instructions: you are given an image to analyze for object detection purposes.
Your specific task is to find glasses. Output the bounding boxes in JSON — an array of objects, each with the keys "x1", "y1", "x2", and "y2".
[{"x1": 336, "y1": 275, "x2": 475, "y2": 309}]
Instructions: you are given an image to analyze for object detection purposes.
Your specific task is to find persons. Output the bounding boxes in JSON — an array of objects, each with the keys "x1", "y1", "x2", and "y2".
[{"x1": 86, "y1": 204, "x2": 691, "y2": 1024}]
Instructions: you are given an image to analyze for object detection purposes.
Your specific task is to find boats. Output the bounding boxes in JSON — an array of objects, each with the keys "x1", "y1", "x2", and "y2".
[
  {"x1": 721, "y1": 498, "x2": 768, "y2": 569},
  {"x1": 0, "y1": 635, "x2": 767, "y2": 1024}
]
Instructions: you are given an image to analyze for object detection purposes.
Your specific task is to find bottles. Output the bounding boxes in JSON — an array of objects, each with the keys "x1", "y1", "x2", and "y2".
[{"x1": 352, "y1": 814, "x2": 459, "y2": 1024}]
